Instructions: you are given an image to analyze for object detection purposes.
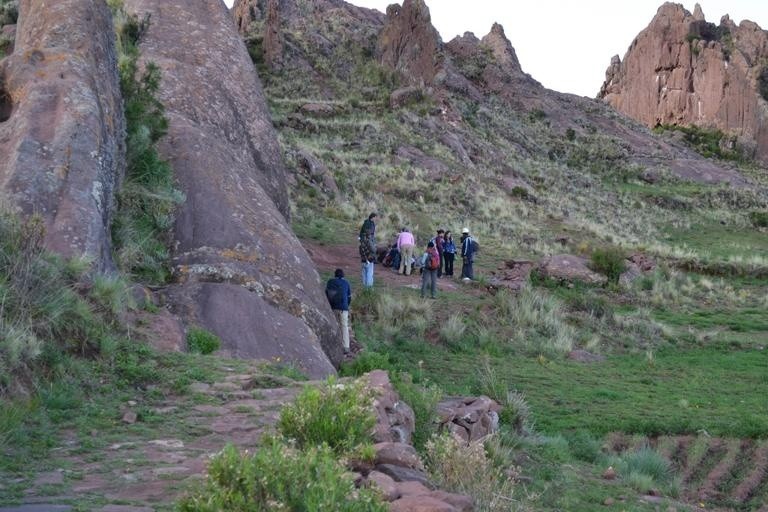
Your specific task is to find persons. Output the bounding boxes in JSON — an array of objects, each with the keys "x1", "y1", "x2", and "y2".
[
  {"x1": 359, "y1": 230, "x2": 378, "y2": 287},
  {"x1": 361, "y1": 212, "x2": 377, "y2": 242},
  {"x1": 324, "y1": 269, "x2": 352, "y2": 355},
  {"x1": 377, "y1": 225, "x2": 480, "y2": 299}
]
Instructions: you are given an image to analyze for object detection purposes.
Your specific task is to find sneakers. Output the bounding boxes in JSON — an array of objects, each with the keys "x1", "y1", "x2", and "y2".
[{"x1": 344, "y1": 347, "x2": 350, "y2": 353}]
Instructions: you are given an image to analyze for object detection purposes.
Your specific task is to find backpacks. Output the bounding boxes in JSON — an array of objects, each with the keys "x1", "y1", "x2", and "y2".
[
  {"x1": 471, "y1": 241, "x2": 480, "y2": 253},
  {"x1": 426, "y1": 253, "x2": 437, "y2": 271},
  {"x1": 324, "y1": 279, "x2": 344, "y2": 309}
]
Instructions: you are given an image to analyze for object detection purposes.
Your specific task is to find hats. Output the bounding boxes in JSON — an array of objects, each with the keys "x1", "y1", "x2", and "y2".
[{"x1": 462, "y1": 228, "x2": 469, "y2": 234}]
